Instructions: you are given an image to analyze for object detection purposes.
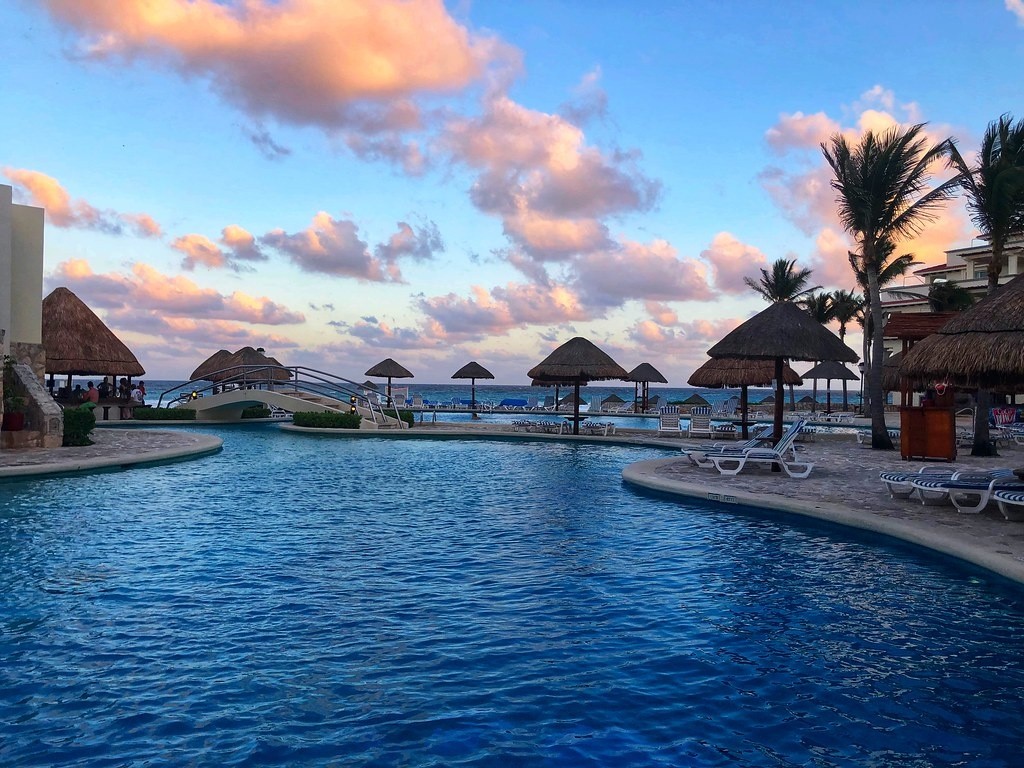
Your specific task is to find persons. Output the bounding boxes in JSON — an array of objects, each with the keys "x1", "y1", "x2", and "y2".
[
  {"x1": 83, "y1": 381, "x2": 99, "y2": 412},
  {"x1": 119, "y1": 378, "x2": 147, "y2": 419},
  {"x1": 97, "y1": 377, "x2": 113, "y2": 398}
]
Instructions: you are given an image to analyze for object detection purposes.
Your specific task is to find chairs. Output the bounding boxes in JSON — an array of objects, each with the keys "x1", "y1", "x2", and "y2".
[{"x1": 404, "y1": 403, "x2": 1024, "y2": 522}]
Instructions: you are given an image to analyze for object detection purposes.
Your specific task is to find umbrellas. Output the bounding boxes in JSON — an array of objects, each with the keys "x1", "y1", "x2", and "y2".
[
  {"x1": 189, "y1": 346, "x2": 295, "y2": 394},
  {"x1": 451, "y1": 362, "x2": 495, "y2": 408},
  {"x1": 621, "y1": 363, "x2": 668, "y2": 413},
  {"x1": 687, "y1": 355, "x2": 803, "y2": 440},
  {"x1": 528, "y1": 337, "x2": 629, "y2": 435},
  {"x1": 42, "y1": 288, "x2": 145, "y2": 390},
  {"x1": 532, "y1": 380, "x2": 587, "y2": 411},
  {"x1": 365, "y1": 359, "x2": 414, "y2": 407},
  {"x1": 802, "y1": 360, "x2": 860, "y2": 412},
  {"x1": 707, "y1": 301, "x2": 860, "y2": 472},
  {"x1": 881, "y1": 273, "x2": 1024, "y2": 407}
]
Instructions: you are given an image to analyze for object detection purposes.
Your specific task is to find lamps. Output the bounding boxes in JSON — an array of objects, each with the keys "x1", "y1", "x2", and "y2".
[
  {"x1": 351, "y1": 396, "x2": 356, "y2": 404},
  {"x1": 192, "y1": 391, "x2": 197, "y2": 400},
  {"x1": 351, "y1": 406, "x2": 356, "y2": 415}
]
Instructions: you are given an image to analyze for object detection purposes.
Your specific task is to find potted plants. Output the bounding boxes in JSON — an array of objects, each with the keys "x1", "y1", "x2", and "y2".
[{"x1": 3, "y1": 397, "x2": 29, "y2": 431}]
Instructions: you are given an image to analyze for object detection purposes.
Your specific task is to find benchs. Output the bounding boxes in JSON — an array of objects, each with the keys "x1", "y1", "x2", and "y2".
[{"x1": 93, "y1": 404, "x2": 126, "y2": 419}]
